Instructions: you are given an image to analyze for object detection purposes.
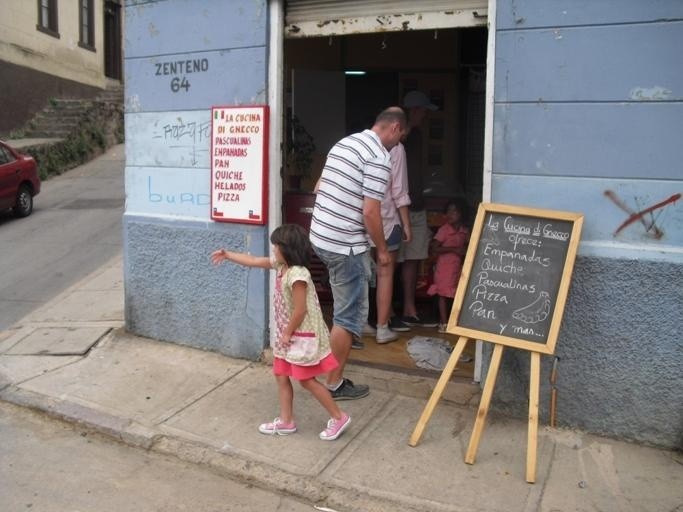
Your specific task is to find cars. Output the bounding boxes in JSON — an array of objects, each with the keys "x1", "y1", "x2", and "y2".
[{"x1": 0, "y1": 141, "x2": 41, "y2": 218}]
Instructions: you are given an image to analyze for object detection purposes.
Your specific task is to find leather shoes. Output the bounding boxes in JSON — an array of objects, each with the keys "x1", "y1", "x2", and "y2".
[
  {"x1": 325, "y1": 379, "x2": 370, "y2": 401},
  {"x1": 349, "y1": 339, "x2": 364, "y2": 350}
]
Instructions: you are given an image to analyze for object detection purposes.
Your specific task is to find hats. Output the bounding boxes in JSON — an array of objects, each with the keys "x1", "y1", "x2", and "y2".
[{"x1": 403, "y1": 91, "x2": 439, "y2": 111}]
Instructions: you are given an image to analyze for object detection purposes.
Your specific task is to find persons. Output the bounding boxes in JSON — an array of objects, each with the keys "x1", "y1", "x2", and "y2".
[
  {"x1": 309, "y1": 106, "x2": 411, "y2": 401},
  {"x1": 359, "y1": 125, "x2": 410, "y2": 344},
  {"x1": 430, "y1": 196, "x2": 469, "y2": 335},
  {"x1": 210, "y1": 224, "x2": 351, "y2": 441},
  {"x1": 376, "y1": 88, "x2": 440, "y2": 332}
]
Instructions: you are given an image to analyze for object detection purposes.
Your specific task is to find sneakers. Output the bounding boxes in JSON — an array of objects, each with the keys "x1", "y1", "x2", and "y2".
[
  {"x1": 389, "y1": 316, "x2": 408, "y2": 331},
  {"x1": 376, "y1": 326, "x2": 397, "y2": 344},
  {"x1": 259, "y1": 419, "x2": 296, "y2": 435},
  {"x1": 319, "y1": 413, "x2": 350, "y2": 441},
  {"x1": 438, "y1": 323, "x2": 450, "y2": 333},
  {"x1": 361, "y1": 324, "x2": 377, "y2": 336},
  {"x1": 400, "y1": 315, "x2": 439, "y2": 327}
]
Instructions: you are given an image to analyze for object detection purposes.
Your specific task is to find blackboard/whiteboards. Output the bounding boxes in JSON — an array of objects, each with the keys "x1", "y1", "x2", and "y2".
[{"x1": 445, "y1": 203, "x2": 585, "y2": 355}]
